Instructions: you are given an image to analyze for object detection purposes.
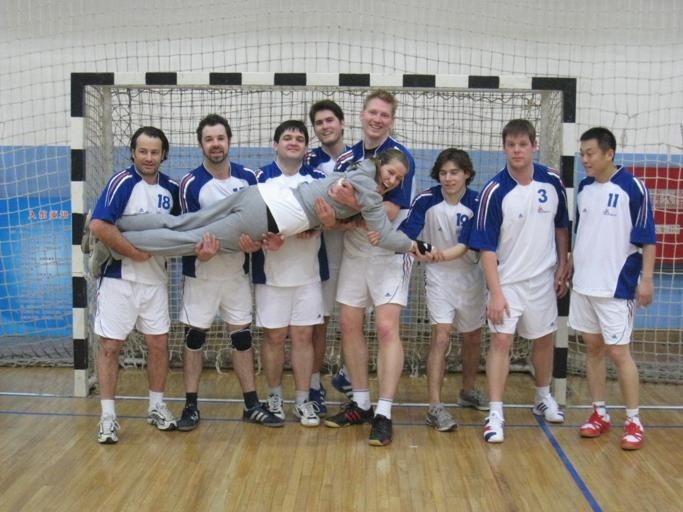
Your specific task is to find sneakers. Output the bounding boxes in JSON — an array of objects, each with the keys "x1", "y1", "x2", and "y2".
[
  {"x1": 88, "y1": 231, "x2": 111, "y2": 279},
  {"x1": 579, "y1": 410, "x2": 610, "y2": 437},
  {"x1": 178, "y1": 407, "x2": 200, "y2": 431},
  {"x1": 82, "y1": 209, "x2": 93, "y2": 254},
  {"x1": 243, "y1": 402, "x2": 284, "y2": 428},
  {"x1": 621, "y1": 417, "x2": 643, "y2": 449},
  {"x1": 267, "y1": 392, "x2": 285, "y2": 420},
  {"x1": 368, "y1": 412, "x2": 392, "y2": 446},
  {"x1": 533, "y1": 393, "x2": 564, "y2": 423},
  {"x1": 146, "y1": 402, "x2": 177, "y2": 430},
  {"x1": 425, "y1": 386, "x2": 504, "y2": 445},
  {"x1": 292, "y1": 368, "x2": 374, "y2": 428},
  {"x1": 97, "y1": 413, "x2": 122, "y2": 443}
]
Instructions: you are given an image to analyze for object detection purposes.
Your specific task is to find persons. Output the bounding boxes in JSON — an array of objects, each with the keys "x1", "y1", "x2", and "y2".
[
  {"x1": 89, "y1": 126, "x2": 181, "y2": 443},
  {"x1": 80, "y1": 150, "x2": 442, "y2": 279},
  {"x1": 176, "y1": 114, "x2": 284, "y2": 432},
  {"x1": 298, "y1": 99, "x2": 359, "y2": 418},
  {"x1": 561, "y1": 127, "x2": 657, "y2": 451},
  {"x1": 315, "y1": 89, "x2": 416, "y2": 448},
  {"x1": 251, "y1": 120, "x2": 330, "y2": 428},
  {"x1": 366, "y1": 148, "x2": 490, "y2": 431},
  {"x1": 467, "y1": 118, "x2": 569, "y2": 443}
]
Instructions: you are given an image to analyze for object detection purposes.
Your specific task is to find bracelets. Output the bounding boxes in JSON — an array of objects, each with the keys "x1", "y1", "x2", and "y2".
[
  {"x1": 325, "y1": 220, "x2": 343, "y2": 229},
  {"x1": 416, "y1": 239, "x2": 432, "y2": 252},
  {"x1": 443, "y1": 247, "x2": 457, "y2": 259}
]
